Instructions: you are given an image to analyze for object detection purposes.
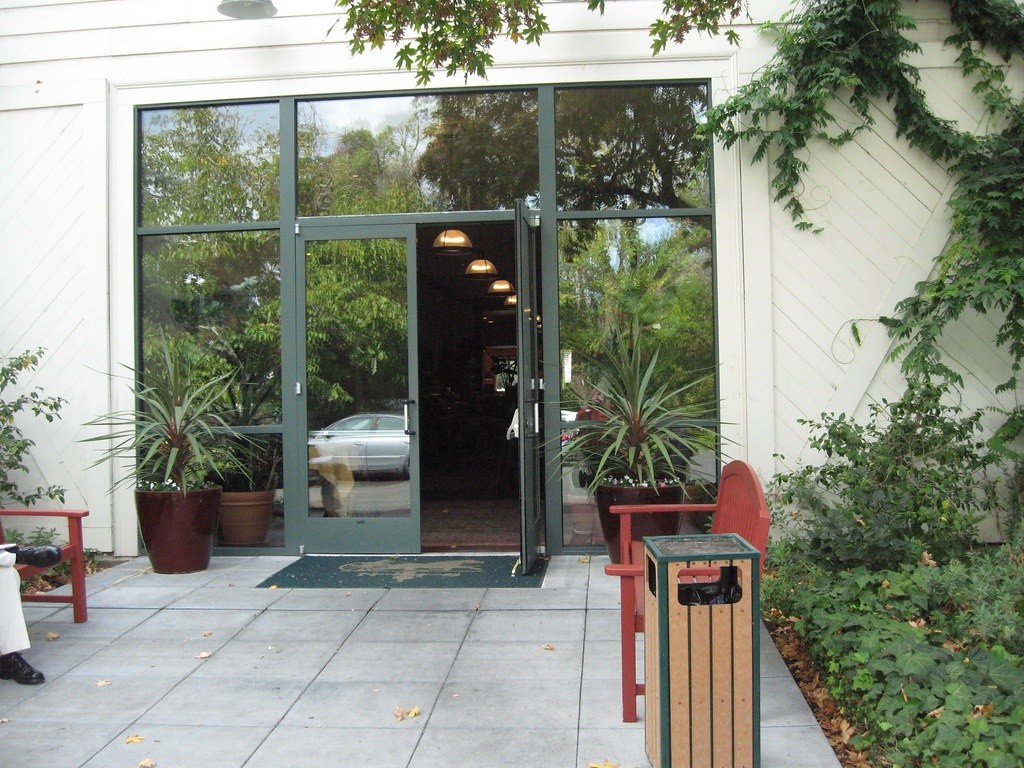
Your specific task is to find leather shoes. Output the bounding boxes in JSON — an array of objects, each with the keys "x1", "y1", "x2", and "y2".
[
  {"x1": 0, "y1": 652, "x2": 45, "y2": 684},
  {"x1": 6, "y1": 545, "x2": 62, "y2": 567}
]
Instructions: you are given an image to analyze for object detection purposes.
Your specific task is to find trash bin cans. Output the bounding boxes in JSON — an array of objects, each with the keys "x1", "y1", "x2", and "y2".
[{"x1": 643, "y1": 533, "x2": 761, "y2": 768}]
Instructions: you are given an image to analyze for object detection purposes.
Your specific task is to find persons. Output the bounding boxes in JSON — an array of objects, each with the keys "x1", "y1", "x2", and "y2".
[{"x1": 0, "y1": 543, "x2": 62, "y2": 684}]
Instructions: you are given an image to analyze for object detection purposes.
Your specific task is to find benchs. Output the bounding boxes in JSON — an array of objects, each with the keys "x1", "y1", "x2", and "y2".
[
  {"x1": 604, "y1": 462, "x2": 772, "y2": 722},
  {"x1": 0, "y1": 509, "x2": 89, "y2": 624}
]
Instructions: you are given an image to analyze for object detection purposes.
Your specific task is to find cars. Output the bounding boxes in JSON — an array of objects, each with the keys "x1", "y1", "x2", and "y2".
[
  {"x1": 562, "y1": 428, "x2": 587, "y2": 466},
  {"x1": 306, "y1": 412, "x2": 410, "y2": 487}
]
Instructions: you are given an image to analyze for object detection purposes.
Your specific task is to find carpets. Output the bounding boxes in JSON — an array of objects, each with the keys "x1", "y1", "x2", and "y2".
[{"x1": 255, "y1": 554, "x2": 552, "y2": 589}]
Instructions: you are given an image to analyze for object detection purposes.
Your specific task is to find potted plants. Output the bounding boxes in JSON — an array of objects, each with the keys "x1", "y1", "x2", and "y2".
[
  {"x1": 196, "y1": 323, "x2": 283, "y2": 548},
  {"x1": 535, "y1": 305, "x2": 743, "y2": 564},
  {"x1": 74, "y1": 320, "x2": 268, "y2": 576}
]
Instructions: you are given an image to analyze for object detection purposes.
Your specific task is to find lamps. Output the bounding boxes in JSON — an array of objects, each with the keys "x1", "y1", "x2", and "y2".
[
  {"x1": 503, "y1": 294, "x2": 517, "y2": 310},
  {"x1": 430, "y1": 140, "x2": 473, "y2": 257},
  {"x1": 217, "y1": 0, "x2": 278, "y2": 20},
  {"x1": 463, "y1": 222, "x2": 502, "y2": 282},
  {"x1": 486, "y1": 278, "x2": 516, "y2": 298}
]
